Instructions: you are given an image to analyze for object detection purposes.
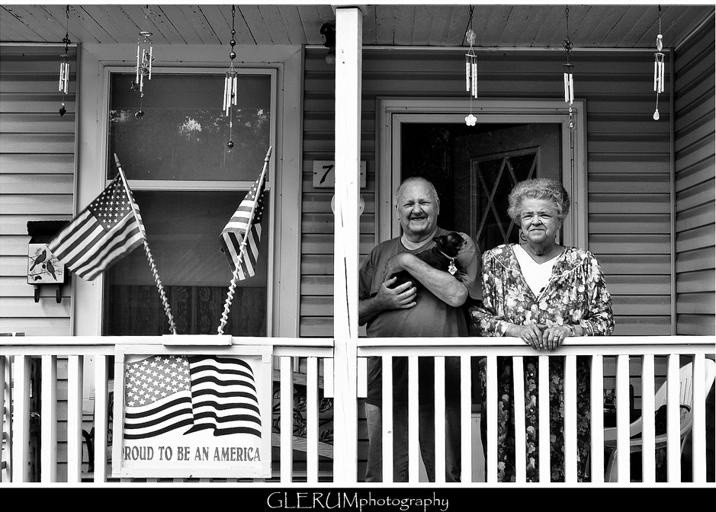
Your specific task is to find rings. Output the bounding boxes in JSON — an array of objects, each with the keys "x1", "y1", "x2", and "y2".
[{"x1": 554, "y1": 334, "x2": 559, "y2": 338}]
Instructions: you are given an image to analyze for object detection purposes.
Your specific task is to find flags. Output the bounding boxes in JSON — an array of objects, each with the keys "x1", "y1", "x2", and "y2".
[
  {"x1": 119, "y1": 352, "x2": 268, "y2": 442},
  {"x1": 217, "y1": 164, "x2": 266, "y2": 284},
  {"x1": 46, "y1": 168, "x2": 147, "y2": 282}
]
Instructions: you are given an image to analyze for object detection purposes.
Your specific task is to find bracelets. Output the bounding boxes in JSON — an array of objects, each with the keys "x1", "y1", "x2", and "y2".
[{"x1": 563, "y1": 321, "x2": 577, "y2": 337}]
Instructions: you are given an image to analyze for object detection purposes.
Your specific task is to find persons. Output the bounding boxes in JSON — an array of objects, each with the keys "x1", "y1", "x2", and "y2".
[
  {"x1": 462, "y1": 175, "x2": 619, "y2": 482},
  {"x1": 359, "y1": 176, "x2": 485, "y2": 482}
]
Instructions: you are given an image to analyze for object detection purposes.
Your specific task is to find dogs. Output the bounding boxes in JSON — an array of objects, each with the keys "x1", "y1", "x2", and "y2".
[{"x1": 360, "y1": 232, "x2": 473, "y2": 303}]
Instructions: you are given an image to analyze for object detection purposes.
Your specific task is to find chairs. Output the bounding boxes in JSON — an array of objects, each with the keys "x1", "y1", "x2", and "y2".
[{"x1": 603, "y1": 359, "x2": 716, "y2": 481}]
